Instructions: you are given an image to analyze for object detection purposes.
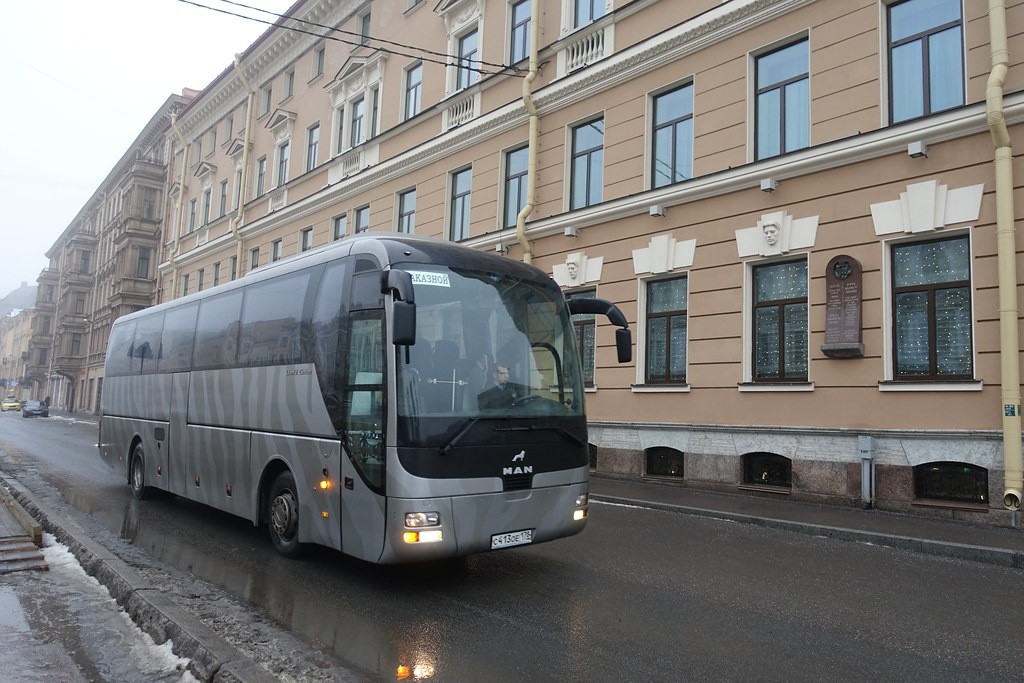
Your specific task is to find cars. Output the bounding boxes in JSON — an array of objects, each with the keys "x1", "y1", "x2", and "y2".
[
  {"x1": 22, "y1": 400, "x2": 51, "y2": 418},
  {"x1": 21, "y1": 401, "x2": 27, "y2": 407},
  {"x1": 1, "y1": 394, "x2": 22, "y2": 412}
]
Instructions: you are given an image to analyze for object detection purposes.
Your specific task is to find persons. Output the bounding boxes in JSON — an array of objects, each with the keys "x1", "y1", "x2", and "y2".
[{"x1": 478, "y1": 362, "x2": 520, "y2": 410}]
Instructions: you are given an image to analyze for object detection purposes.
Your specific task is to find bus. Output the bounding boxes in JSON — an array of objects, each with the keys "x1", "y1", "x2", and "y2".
[{"x1": 96, "y1": 229, "x2": 634, "y2": 566}]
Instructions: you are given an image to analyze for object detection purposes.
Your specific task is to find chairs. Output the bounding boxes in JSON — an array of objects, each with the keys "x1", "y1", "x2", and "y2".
[
  {"x1": 433, "y1": 340, "x2": 461, "y2": 381},
  {"x1": 412, "y1": 338, "x2": 432, "y2": 370}
]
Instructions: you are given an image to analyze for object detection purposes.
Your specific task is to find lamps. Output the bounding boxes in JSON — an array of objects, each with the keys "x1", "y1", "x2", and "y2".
[
  {"x1": 496, "y1": 243, "x2": 509, "y2": 254},
  {"x1": 908, "y1": 141, "x2": 928, "y2": 159},
  {"x1": 760, "y1": 179, "x2": 779, "y2": 193},
  {"x1": 564, "y1": 228, "x2": 578, "y2": 237},
  {"x1": 650, "y1": 205, "x2": 666, "y2": 218}
]
